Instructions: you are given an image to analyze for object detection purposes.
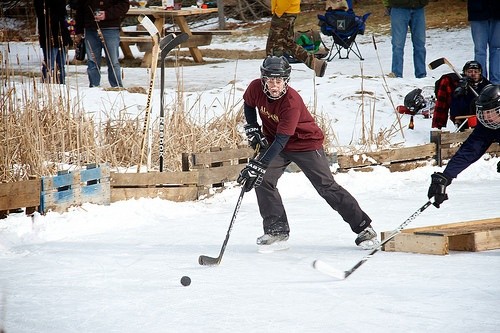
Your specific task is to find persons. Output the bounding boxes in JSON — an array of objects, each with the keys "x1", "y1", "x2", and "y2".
[
  {"x1": 75, "y1": 0, "x2": 130, "y2": 88},
  {"x1": 467, "y1": 0, "x2": 500, "y2": 85},
  {"x1": 266, "y1": 0, "x2": 327, "y2": 78},
  {"x1": 33, "y1": 0, "x2": 74, "y2": 85},
  {"x1": 432, "y1": 60, "x2": 494, "y2": 132},
  {"x1": 236, "y1": 55, "x2": 378, "y2": 247},
  {"x1": 387, "y1": 0, "x2": 430, "y2": 79},
  {"x1": 427, "y1": 84, "x2": 500, "y2": 208}
]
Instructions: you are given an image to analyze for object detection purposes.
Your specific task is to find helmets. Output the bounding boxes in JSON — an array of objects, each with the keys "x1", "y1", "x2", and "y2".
[
  {"x1": 474, "y1": 83, "x2": 500, "y2": 122},
  {"x1": 260, "y1": 55, "x2": 291, "y2": 90},
  {"x1": 405, "y1": 89, "x2": 424, "y2": 112},
  {"x1": 463, "y1": 61, "x2": 482, "y2": 82}
]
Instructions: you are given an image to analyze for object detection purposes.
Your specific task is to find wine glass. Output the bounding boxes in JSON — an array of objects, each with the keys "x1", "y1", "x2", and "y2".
[
  {"x1": 138, "y1": 0, "x2": 147, "y2": 9},
  {"x1": 94, "y1": 11, "x2": 101, "y2": 21},
  {"x1": 196, "y1": 0, "x2": 204, "y2": 9}
]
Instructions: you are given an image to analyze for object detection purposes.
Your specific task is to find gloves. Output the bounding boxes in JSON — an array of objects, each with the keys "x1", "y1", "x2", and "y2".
[
  {"x1": 427, "y1": 172, "x2": 451, "y2": 208},
  {"x1": 497, "y1": 161, "x2": 500, "y2": 173},
  {"x1": 244, "y1": 124, "x2": 268, "y2": 152},
  {"x1": 460, "y1": 76, "x2": 475, "y2": 95},
  {"x1": 237, "y1": 159, "x2": 267, "y2": 192}
]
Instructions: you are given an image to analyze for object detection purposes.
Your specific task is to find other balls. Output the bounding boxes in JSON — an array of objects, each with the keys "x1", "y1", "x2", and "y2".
[{"x1": 181, "y1": 276, "x2": 191, "y2": 286}]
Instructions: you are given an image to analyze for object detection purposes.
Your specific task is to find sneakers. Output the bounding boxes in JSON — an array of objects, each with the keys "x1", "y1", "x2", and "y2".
[
  {"x1": 355, "y1": 225, "x2": 379, "y2": 248},
  {"x1": 257, "y1": 230, "x2": 290, "y2": 252}
]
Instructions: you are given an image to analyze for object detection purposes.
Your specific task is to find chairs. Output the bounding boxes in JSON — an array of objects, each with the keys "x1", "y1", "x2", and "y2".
[{"x1": 317, "y1": 7, "x2": 371, "y2": 62}]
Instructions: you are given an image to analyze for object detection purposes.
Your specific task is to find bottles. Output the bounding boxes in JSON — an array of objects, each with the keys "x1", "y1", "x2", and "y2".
[{"x1": 408, "y1": 116, "x2": 414, "y2": 130}]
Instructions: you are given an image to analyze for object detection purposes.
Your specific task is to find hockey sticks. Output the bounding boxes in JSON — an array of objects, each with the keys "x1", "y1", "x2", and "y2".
[
  {"x1": 159, "y1": 32, "x2": 190, "y2": 172},
  {"x1": 137, "y1": 12, "x2": 162, "y2": 173},
  {"x1": 146, "y1": 33, "x2": 176, "y2": 172},
  {"x1": 312, "y1": 194, "x2": 436, "y2": 281},
  {"x1": 198, "y1": 177, "x2": 247, "y2": 266},
  {"x1": 428, "y1": 57, "x2": 479, "y2": 97}
]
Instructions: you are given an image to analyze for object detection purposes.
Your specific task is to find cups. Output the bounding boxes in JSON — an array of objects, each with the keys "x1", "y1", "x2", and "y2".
[{"x1": 174, "y1": 0, "x2": 182, "y2": 9}]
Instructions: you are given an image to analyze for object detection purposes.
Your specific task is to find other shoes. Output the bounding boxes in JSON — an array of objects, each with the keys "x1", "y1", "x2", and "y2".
[{"x1": 310, "y1": 58, "x2": 327, "y2": 77}]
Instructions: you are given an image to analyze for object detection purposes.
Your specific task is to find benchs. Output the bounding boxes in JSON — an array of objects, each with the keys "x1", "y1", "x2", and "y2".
[{"x1": 68, "y1": 30, "x2": 232, "y2": 43}]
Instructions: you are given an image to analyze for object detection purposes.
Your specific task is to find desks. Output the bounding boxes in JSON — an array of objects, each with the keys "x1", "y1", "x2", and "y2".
[{"x1": 70, "y1": 7, "x2": 219, "y2": 68}]
